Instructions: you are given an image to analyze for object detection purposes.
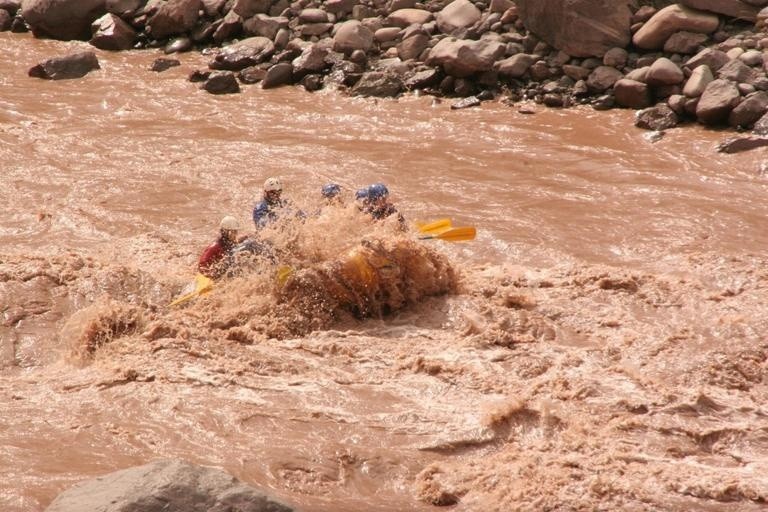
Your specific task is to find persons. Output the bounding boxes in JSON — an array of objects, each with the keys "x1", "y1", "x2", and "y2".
[
  {"x1": 318, "y1": 183, "x2": 346, "y2": 208},
  {"x1": 252, "y1": 177, "x2": 320, "y2": 231},
  {"x1": 355, "y1": 189, "x2": 371, "y2": 214},
  {"x1": 197, "y1": 216, "x2": 275, "y2": 283},
  {"x1": 364, "y1": 183, "x2": 409, "y2": 232}
]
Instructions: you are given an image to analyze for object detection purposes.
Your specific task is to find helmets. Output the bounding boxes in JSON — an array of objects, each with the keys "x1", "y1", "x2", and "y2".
[
  {"x1": 354, "y1": 183, "x2": 390, "y2": 202},
  {"x1": 321, "y1": 183, "x2": 340, "y2": 196},
  {"x1": 263, "y1": 176, "x2": 282, "y2": 191},
  {"x1": 218, "y1": 216, "x2": 241, "y2": 229}
]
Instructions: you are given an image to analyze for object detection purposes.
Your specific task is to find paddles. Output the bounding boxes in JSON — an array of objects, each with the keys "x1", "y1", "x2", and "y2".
[
  {"x1": 419, "y1": 219, "x2": 453, "y2": 233},
  {"x1": 418, "y1": 228, "x2": 476, "y2": 241},
  {"x1": 171, "y1": 279, "x2": 213, "y2": 306}
]
